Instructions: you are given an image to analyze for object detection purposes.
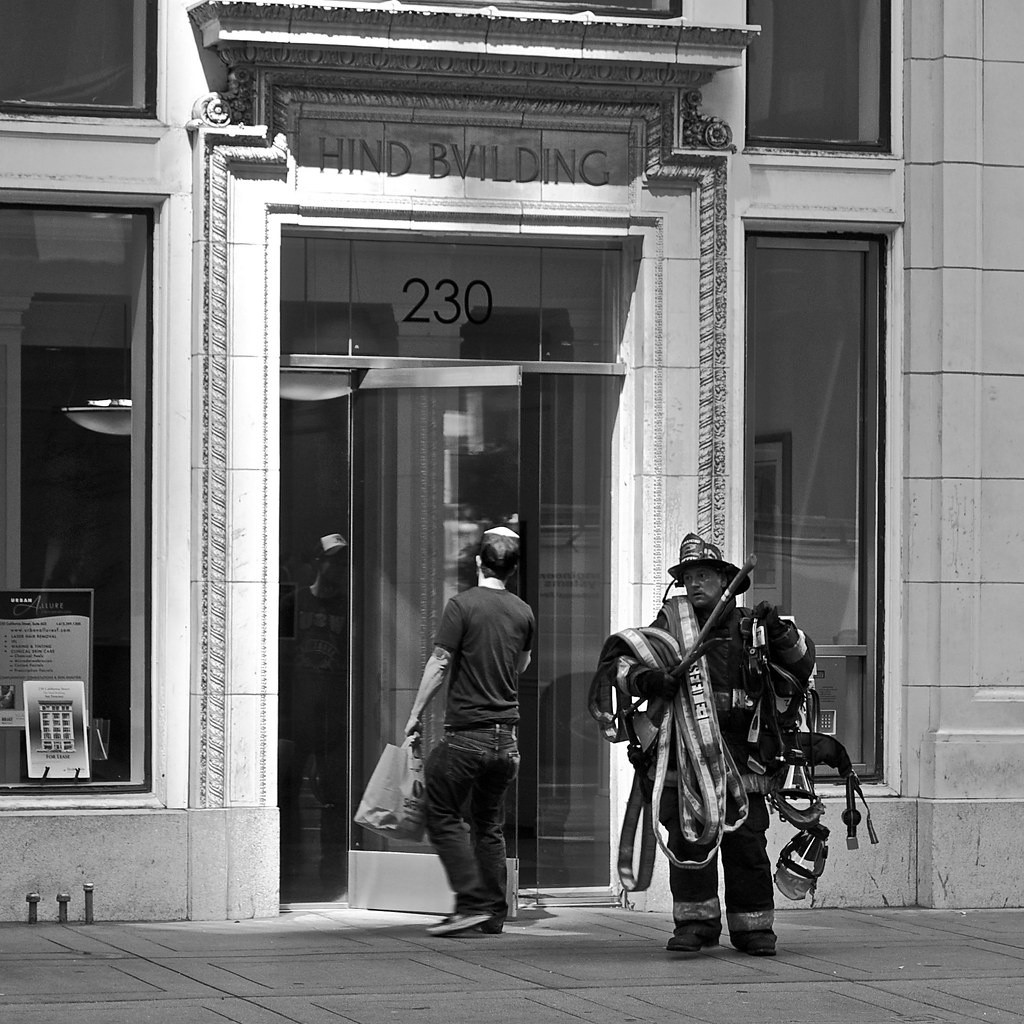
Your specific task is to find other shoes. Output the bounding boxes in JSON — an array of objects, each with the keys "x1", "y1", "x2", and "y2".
[
  {"x1": 425, "y1": 913, "x2": 493, "y2": 935},
  {"x1": 748, "y1": 947, "x2": 777, "y2": 956},
  {"x1": 472, "y1": 922, "x2": 502, "y2": 935},
  {"x1": 666, "y1": 935, "x2": 719, "y2": 951}
]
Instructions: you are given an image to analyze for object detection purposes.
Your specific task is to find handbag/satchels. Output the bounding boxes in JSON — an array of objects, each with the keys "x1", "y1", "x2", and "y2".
[{"x1": 354, "y1": 737, "x2": 428, "y2": 843}]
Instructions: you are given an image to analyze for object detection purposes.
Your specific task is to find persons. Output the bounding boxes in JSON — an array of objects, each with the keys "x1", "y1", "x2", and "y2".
[
  {"x1": 617, "y1": 531, "x2": 815, "y2": 957},
  {"x1": 400, "y1": 525, "x2": 537, "y2": 937},
  {"x1": 279, "y1": 513, "x2": 528, "y2": 887}
]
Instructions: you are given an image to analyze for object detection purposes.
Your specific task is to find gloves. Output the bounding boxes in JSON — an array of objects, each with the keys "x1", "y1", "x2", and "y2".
[
  {"x1": 648, "y1": 667, "x2": 681, "y2": 703},
  {"x1": 750, "y1": 600, "x2": 776, "y2": 627}
]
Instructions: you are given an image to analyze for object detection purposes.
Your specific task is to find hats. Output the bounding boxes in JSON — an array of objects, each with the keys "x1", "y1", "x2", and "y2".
[
  {"x1": 668, "y1": 533, "x2": 750, "y2": 596},
  {"x1": 311, "y1": 533, "x2": 348, "y2": 558},
  {"x1": 480, "y1": 527, "x2": 520, "y2": 570}
]
